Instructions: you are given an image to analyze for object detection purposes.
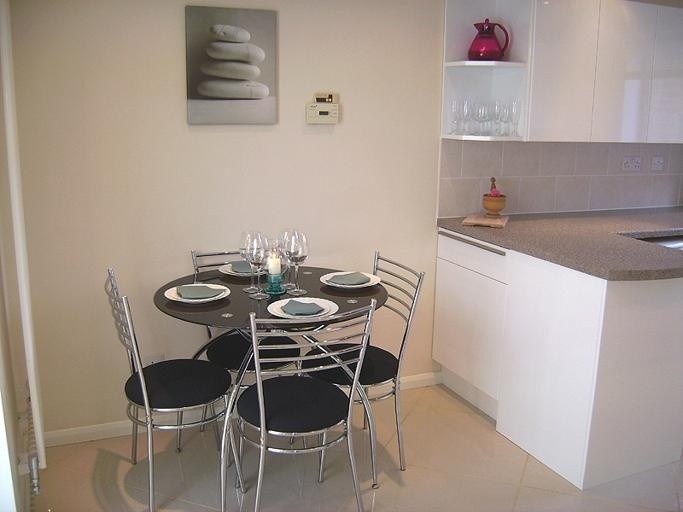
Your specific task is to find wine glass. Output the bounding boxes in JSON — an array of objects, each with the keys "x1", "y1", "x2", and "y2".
[
  {"x1": 241, "y1": 230, "x2": 309, "y2": 300},
  {"x1": 448, "y1": 95, "x2": 521, "y2": 139}
]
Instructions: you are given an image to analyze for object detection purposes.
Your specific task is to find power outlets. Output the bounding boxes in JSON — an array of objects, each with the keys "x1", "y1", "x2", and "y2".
[{"x1": 622, "y1": 156, "x2": 641, "y2": 172}]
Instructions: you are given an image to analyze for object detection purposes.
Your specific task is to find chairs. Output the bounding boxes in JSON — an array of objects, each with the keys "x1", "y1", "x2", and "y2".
[
  {"x1": 300, "y1": 249, "x2": 424, "y2": 483},
  {"x1": 190, "y1": 249, "x2": 301, "y2": 432},
  {"x1": 235, "y1": 298, "x2": 379, "y2": 512},
  {"x1": 105, "y1": 267, "x2": 247, "y2": 512}
]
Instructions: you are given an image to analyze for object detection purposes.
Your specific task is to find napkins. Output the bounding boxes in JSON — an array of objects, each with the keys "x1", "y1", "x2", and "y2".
[
  {"x1": 176, "y1": 285, "x2": 226, "y2": 300},
  {"x1": 230, "y1": 261, "x2": 258, "y2": 273},
  {"x1": 327, "y1": 270, "x2": 370, "y2": 285},
  {"x1": 281, "y1": 300, "x2": 324, "y2": 316}
]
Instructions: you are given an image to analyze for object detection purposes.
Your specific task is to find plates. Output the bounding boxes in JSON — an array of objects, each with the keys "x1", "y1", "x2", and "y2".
[
  {"x1": 267, "y1": 297, "x2": 339, "y2": 321},
  {"x1": 164, "y1": 284, "x2": 231, "y2": 305},
  {"x1": 218, "y1": 263, "x2": 266, "y2": 277},
  {"x1": 319, "y1": 271, "x2": 381, "y2": 289}
]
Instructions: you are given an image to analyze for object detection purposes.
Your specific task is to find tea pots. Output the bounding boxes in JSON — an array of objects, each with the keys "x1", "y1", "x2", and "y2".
[{"x1": 467, "y1": 19, "x2": 509, "y2": 61}]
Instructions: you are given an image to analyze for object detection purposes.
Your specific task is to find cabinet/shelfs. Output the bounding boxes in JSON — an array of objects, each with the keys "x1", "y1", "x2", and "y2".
[
  {"x1": 432, "y1": 226, "x2": 509, "y2": 422},
  {"x1": 646, "y1": 0, "x2": 682, "y2": 143},
  {"x1": 440, "y1": 1, "x2": 533, "y2": 141},
  {"x1": 528, "y1": 0, "x2": 658, "y2": 143}
]
